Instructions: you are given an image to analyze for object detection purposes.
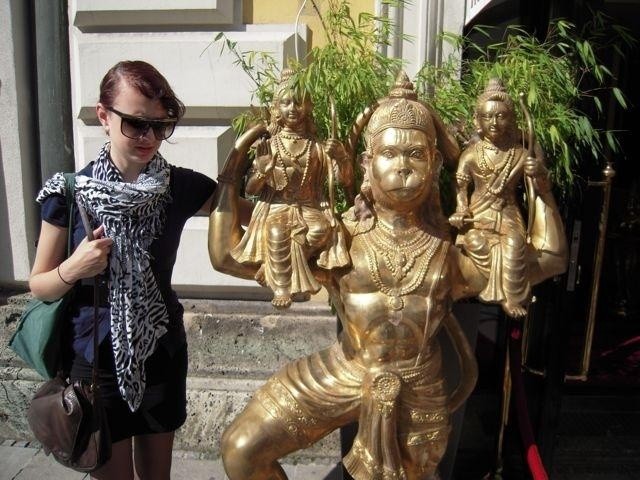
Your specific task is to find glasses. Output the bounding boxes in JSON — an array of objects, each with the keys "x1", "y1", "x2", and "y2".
[{"x1": 106, "y1": 107, "x2": 178, "y2": 140}]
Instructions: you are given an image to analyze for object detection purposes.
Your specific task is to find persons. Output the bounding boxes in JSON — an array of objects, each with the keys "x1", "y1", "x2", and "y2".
[
  {"x1": 28, "y1": 59, "x2": 258, "y2": 479},
  {"x1": 447, "y1": 76, "x2": 551, "y2": 318},
  {"x1": 243, "y1": 75, "x2": 351, "y2": 309},
  {"x1": 207, "y1": 73, "x2": 568, "y2": 478}
]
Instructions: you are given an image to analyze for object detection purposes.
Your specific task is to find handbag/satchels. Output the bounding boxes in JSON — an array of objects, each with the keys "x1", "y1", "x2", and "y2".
[
  {"x1": 26, "y1": 376, "x2": 112, "y2": 472},
  {"x1": 6, "y1": 298, "x2": 64, "y2": 379}
]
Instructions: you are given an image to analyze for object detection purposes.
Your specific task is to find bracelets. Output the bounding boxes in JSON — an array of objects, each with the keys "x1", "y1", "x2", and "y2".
[{"x1": 57, "y1": 265, "x2": 74, "y2": 286}]
